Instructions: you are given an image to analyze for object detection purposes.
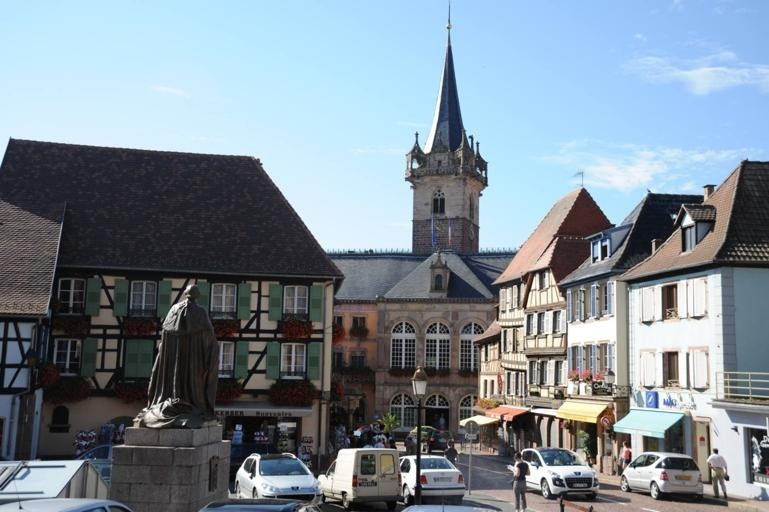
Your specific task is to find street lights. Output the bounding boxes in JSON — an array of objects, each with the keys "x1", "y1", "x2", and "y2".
[{"x1": 411, "y1": 366, "x2": 428, "y2": 504}]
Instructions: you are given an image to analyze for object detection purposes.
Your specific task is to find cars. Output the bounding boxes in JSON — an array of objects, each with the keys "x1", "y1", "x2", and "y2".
[
  {"x1": 406, "y1": 426, "x2": 454, "y2": 450},
  {"x1": 511, "y1": 447, "x2": 600, "y2": 500},
  {"x1": 620, "y1": 451, "x2": 703, "y2": 500},
  {"x1": 399, "y1": 455, "x2": 466, "y2": 506}
]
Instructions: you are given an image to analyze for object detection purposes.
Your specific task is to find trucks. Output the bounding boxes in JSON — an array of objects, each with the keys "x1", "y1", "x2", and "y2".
[{"x1": 316, "y1": 447, "x2": 398, "y2": 511}]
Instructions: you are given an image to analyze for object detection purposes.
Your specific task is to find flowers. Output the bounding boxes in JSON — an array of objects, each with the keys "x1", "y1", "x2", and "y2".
[{"x1": 566, "y1": 368, "x2": 608, "y2": 385}]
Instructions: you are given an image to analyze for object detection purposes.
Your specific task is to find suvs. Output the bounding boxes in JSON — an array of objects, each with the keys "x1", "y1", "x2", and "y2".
[{"x1": 235, "y1": 453, "x2": 318, "y2": 499}]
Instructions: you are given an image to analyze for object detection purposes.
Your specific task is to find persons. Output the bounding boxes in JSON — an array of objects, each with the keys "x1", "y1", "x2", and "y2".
[
  {"x1": 512, "y1": 452, "x2": 530, "y2": 512},
  {"x1": 621, "y1": 441, "x2": 631, "y2": 469},
  {"x1": 706, "y1": 448, "x2": 728, "y2": 498},
  {"x1": 349, "y1": 432, "x2": 458, "y2": 466},
  {"x1": 135, "y1": 284, "x2": 220, "y2": 428}
]
